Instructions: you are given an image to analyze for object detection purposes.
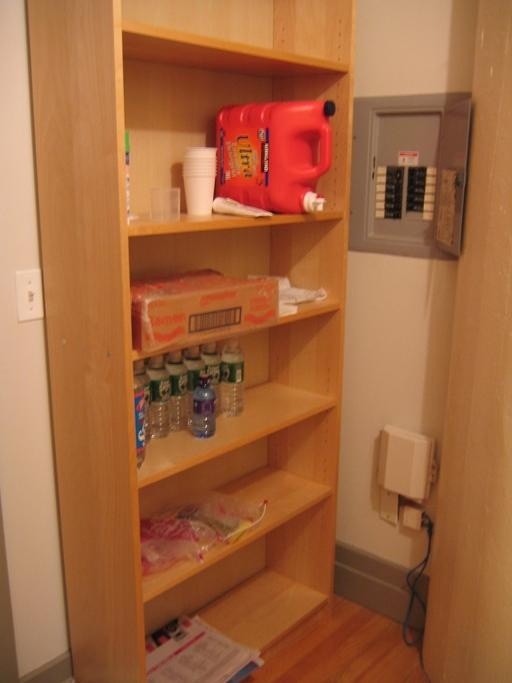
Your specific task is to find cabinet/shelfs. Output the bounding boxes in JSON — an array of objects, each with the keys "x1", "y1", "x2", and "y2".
[{"x1": 25, "y1": 0, "x2": 359, "y2": 683}]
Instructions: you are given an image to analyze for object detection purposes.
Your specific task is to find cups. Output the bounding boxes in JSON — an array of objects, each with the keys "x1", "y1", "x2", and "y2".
[{"x1": 182, "y1": 146, "x2": 218, "y2": 218}]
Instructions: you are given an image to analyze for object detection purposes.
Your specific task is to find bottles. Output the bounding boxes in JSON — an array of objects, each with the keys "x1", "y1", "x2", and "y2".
[{"x1": 134, "y1": 338, "x2": 245, "y2": 466}]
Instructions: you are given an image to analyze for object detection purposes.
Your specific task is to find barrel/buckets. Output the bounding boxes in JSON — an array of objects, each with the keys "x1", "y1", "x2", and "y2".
[{"x1": 215, "y1": 98, "x2": 335, "y2": 215}]
